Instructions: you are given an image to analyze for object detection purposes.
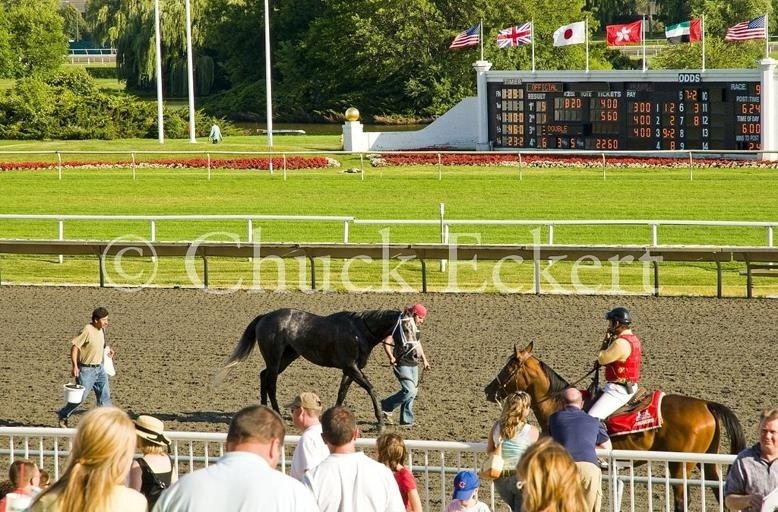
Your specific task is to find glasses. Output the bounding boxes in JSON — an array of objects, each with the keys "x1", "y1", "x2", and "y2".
[
  {"x1": 516, "y1": 480, "x2": 526, "y2": 489},
  {"x1": 291, "y1": 409, "x2": 294, "y2": 413},
  {"x1": 45, "y1": 482, "x2": 51, "y2": 486}
]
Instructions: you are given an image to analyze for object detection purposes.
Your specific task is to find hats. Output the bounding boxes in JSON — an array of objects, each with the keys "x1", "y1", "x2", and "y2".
[
  {"x1": 451, "y1": 469, "x2": 480, "y2": 501},
  {"x1": 409, "y1": 305, "x2": 426, "y2": 316},
  {"x1": 135, "y1": 415, "x2": 172, "y2": 446},
  {"x1": 284, "y1": 391, "x2": 322, "y2": 410}
]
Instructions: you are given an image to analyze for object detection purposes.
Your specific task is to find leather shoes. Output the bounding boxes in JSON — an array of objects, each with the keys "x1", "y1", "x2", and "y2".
[{"x1": 57, "y1": 410, "x2": 67, "y2": 429}]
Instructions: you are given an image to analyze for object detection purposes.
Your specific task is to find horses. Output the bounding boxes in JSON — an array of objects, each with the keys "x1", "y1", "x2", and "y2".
[
  {"x1": 211, "y1": 307, "x2": 417, "y2": 433},
  {"x1": 484, "y1": 340, "x2": 749, "y2": 512}
]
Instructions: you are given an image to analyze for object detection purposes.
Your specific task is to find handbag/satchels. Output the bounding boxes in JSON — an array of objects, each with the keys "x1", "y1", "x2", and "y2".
[
  {"x1": 101, "y1": 327, "x2": 116, "y2": 376},
  {"x1": 480, "y1": 422, "x2": 505, "y2": 479},
  {"x1": 137, "y1": 457, "x2": 167, "y2": 490}
]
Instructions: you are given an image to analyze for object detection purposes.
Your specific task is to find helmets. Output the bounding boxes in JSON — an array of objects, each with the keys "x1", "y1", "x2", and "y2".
[{"x1": 606, "y1": 308, "x2": 632, "y2": 323}]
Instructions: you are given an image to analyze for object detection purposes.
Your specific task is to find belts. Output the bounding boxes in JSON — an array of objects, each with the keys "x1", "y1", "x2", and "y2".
[
  {"x1": 606, "y1": 380, "x2": 636, "y2": 387},
  {"x1": 78, "y1": 361, "x2": 101, "y2": 369}
]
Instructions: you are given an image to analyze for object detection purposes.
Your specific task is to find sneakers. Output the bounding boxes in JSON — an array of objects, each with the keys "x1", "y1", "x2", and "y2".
[
  {"x1": 384, "y1": 409, "x2": 392, "y2": 424},
  {"x1": 401, "y1": 421, "x2": 417, "y2": 426}
]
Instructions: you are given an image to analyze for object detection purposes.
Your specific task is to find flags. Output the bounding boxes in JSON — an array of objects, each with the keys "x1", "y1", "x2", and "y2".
[
  {"x1": 606, "y1": 13, "x2": 646, "y2": 46},
  {"x1": 665, "y1": 13, "x2": 705, "y2": 44},
  {"x1": 553, "y1": 16, "x2": 589, "y2": 47},
  {"x1": 497, "y1": 17, "x2": 535, "y2": 48},
  {"x1": 723, "y1": 10, "x2": 768, "y2": 42},
  {"x1": 448, "y1": 17, "x2": 483, "y2": 48}
]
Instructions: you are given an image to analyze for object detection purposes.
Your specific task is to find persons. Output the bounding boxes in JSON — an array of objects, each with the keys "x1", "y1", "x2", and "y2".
[
  {"x1": 486, "y1": 391, "x2": 542, "y2": 512},
  {"x1": 514, "y1": 436, "x2": 593, "y2": 512},
  {"x1": 209, "y1": 121, "x2": 223, "y2": 144},
  {"x1": 723, "y1": 407, "x2": 778, "y2": 511},
  {"x1": 549, "y1": 388, "x2": 612, "y2": 512},
  {"x1": 0, "y1": 460, "x2": 53, "y2": 511},
  {"x1": 444, "y1": 472, "x2": 490, "y2": 512},
  {"x1": 23, "y1": 405, "x2": 149, "y2": 512},
  {"x1": 586, "y1": 308, "x2": 640, "y2": 450},
  {"x1": 375, "y1": 434, "x2": 422, "y2": 511},
  {"x1": 282, "y1": 392, "x2": 331, "y2": 482},
  {"x1": 130, "y1": 415, "x2": 178, "y2": 511},
  {"x1": 153, "y1": 405, "x2": 321, "y2": 512},
  {"x1": 57, "y1": 307, "x2": 115, "y2": 427},
  {"x1": 380, "y1": 304, "x2": 430, "y2": 425},
  {"x1": 302, "y1": 406, "x2": 407, "y2": 512}
]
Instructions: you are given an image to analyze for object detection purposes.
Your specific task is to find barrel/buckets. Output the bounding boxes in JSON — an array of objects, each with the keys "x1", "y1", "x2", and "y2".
[{"x1": 64, "y1": 384, "x2": 84, "y2": 404}]
